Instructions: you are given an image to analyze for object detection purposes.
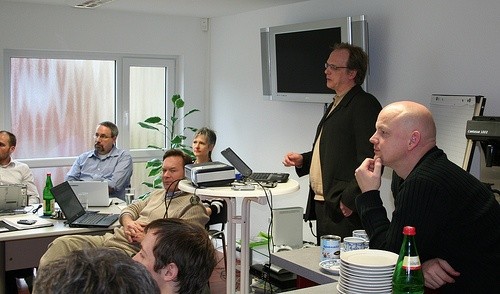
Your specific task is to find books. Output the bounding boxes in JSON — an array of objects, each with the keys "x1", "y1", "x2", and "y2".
[{"x1": 0, "y1": 213, "x2": 54, "y2": 233}]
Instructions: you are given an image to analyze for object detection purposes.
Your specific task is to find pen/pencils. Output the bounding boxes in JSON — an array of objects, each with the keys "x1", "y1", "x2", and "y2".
[{"x1": 32, "y1": 204, "x2": 42, "y2": 214}]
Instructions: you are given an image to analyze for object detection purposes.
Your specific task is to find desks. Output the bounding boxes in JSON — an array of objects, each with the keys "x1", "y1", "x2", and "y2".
[
  {"x1": 0, "y1": 196, "x2": 127, "y2": 294},
  {"x1": 178, "y1": 177, "x2": 300, "y2": 294},
  {"x1": 268, "y1": 242, "x2": 370, "y2": 294}
]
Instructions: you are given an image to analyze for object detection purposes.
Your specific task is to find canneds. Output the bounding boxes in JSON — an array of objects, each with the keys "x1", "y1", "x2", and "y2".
[{"x1": 320, "y1": 235, "x2": 341, "y2": 263}]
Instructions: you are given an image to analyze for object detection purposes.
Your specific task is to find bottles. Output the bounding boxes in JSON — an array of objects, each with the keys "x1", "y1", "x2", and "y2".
[
  {"x1": 43, "y1": 172, "x2": 56, "y2": 216},
  {"x1": 391, "y1": 226, "x2": 425, "y2": 294}
]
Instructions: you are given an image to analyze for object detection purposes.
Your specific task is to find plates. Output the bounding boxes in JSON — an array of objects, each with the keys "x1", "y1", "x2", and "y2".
[
  {"x1": 319, "y1": 259, "x2": 340, "y2": 273},
  {"x1": 336, "y1": 249, "x2": 400, "y2": 294}
]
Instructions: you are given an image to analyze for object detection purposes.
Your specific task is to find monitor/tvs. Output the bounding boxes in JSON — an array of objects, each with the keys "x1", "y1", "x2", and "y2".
[{"x1": 269, "y1": 17, "x2": 352, "y2": 104}]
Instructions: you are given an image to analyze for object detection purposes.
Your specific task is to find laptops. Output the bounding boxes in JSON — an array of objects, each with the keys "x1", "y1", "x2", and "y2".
[
  {"x1": 51, "y1": 181, "x2": 120, "y2": 228},
  {"x1": 221, "y1": 147, "x2": 289, "y2": 183},
  {"x1": 68, "y1": 180, "x2": 113, "y2": 206}
]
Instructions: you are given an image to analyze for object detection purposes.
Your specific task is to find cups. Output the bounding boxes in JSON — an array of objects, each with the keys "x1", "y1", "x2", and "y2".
[
  {"x1": 125, "y1": 187, "x2": 135, "y2": 206},
  {"x1": 79, "y1": 191, "x2": 88, "y2": 209}
]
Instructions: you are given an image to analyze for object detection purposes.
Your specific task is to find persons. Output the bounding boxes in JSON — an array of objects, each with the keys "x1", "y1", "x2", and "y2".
[
  {"x1": 282, "y1": 42, "x2": 385, "y2": 246},
  {"x1": 189, "y1": 127, "x2": 224, "y2": 221},
  {"x1": 354, "y1": 101, "x2": 500, "y2": 294},
  {"x1": 64, "y1": 121, "x2": 134, "y2": 201},
  {"x1": 32, "y1": 149, "x2": 210, "y2": 294},
  {"x1": 30, "y1": 249, "x2": 161, "y2": 294},
  {"x1": 132, "y1": 218, "x2": 218, "y2": 294},
  {"x1": 0, "y1": 131, "x2": 42, "y2": 203}
]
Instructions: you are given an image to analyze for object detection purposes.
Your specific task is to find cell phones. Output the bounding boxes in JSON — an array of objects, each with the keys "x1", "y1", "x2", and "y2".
[{"x1": 18, "y1": 219, "x2": 35, "y2": 224}]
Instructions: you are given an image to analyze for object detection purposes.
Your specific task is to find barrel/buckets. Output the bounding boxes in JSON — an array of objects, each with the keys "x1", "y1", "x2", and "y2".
[
  {"x1": 320, "y1": 235, "x2": 341, "y2": 261},
  {"x1": 353, "y1": 230, "x2": 369, "y2": 249},
  {"x1": 343, "y1": 236, "x2": 366, "y2": 250}
]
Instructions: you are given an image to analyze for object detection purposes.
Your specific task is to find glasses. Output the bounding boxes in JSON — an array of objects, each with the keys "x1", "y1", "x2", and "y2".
[
  {"x1": 325, "y1": 63, "x2": 348, "y2": 71},
  {"x1": 93, "y1": 134, "x2": 113, "y2": 139}
]
